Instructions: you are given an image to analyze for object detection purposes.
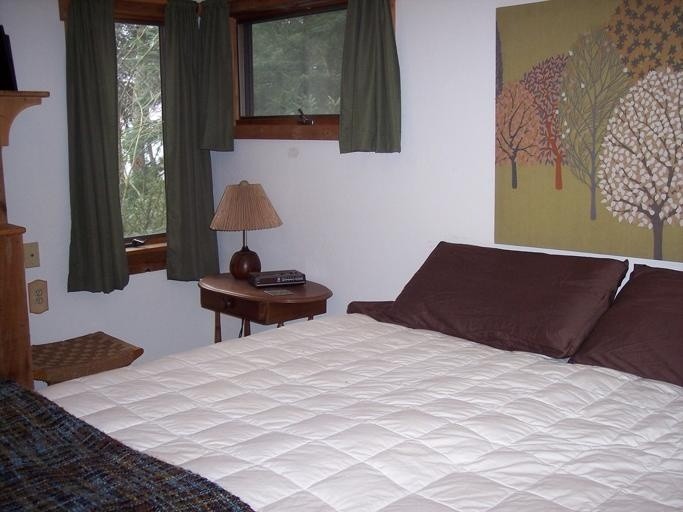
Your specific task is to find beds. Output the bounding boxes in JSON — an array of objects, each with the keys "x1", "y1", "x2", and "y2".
[{"x1": 0, "y1": 312, "x2": 683, "y2": 512}]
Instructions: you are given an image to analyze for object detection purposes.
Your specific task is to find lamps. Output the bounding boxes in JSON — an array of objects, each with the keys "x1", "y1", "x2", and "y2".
[{"x1": 209, "y1": 180, "x2": 283, "y2": 279}]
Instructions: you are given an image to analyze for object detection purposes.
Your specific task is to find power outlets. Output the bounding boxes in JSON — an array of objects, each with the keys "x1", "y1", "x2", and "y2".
[{"x1": 28, "y1": 280, "x2": 49, "y2": 314}]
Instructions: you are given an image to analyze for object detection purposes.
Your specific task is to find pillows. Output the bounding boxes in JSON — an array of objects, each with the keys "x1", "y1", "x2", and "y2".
[
  {"x1": 385, "y1": 241, "x2": 628, "y2": 359},
  {"x1": 567, "y1": 263, "x2": 683, "y2": 386}
]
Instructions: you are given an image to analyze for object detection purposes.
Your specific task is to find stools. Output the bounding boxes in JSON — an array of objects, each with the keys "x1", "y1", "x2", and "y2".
[{"x1": 32, "y1": 332, "x2": 143, "y2": 386}]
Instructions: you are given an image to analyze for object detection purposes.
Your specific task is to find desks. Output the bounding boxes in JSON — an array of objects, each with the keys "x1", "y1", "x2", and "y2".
[{"x1": 197, "y1": 274, "x2": 333, "y2": 344}]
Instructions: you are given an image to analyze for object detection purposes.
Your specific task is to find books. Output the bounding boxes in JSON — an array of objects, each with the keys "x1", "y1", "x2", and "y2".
[{"x1": 248, "y1": 269, "x2": 306, "y2": 287}]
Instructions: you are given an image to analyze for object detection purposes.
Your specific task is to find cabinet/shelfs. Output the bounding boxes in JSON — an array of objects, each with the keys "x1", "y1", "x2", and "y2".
[{"x1": 0, "y1": 91, "x2": 50, "y2": 392}]
